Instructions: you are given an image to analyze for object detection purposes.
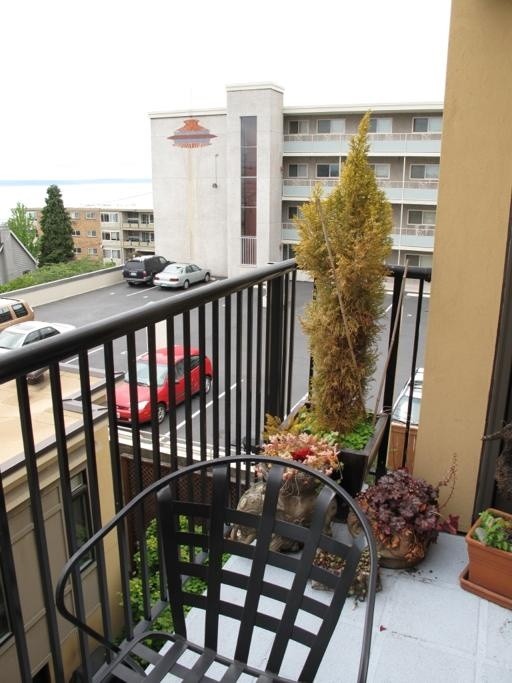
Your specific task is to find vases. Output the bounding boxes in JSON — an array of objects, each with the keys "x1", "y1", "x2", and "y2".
[{"x1": 231, "y1": 480, "x2": 337, "y2": 552}]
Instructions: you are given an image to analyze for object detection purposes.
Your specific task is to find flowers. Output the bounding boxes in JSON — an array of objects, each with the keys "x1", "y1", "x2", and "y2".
[{"x1": 263, "y1": 432, "x2": 342, "y2": 485}]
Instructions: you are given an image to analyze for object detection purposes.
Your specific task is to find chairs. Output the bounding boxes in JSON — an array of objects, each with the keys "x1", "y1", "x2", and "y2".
[{"x1": 55, "y1": 456, "x2": 383, "y2": 683}]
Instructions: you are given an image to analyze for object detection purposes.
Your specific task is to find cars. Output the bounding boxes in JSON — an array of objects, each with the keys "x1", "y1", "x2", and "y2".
[
  {"x1": 0, "y1": 319, "x2": 78, "y2": 360},
  {"x1": 152, "y1": 262, "x2": 212, "y2": 290},
  {"x1": 108, "y1": 342, "x2": 214, "y2": 427}
]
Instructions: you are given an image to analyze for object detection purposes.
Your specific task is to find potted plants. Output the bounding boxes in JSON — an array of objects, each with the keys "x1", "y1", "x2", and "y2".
[
  {"x1": 276, "y1": 107, "x2": 392, "y2": 500},
  {"x1": 347, "y1": 468, "x2": 511, "y2": 612}
]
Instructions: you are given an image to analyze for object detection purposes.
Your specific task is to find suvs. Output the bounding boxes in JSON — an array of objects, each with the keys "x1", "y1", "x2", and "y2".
[
  {"x1": 122, "y1": 255, "x2": 176, "y2": 287},
  {"x1": 0, "y1": 293, "x2": 37, "y2": 334}
]
